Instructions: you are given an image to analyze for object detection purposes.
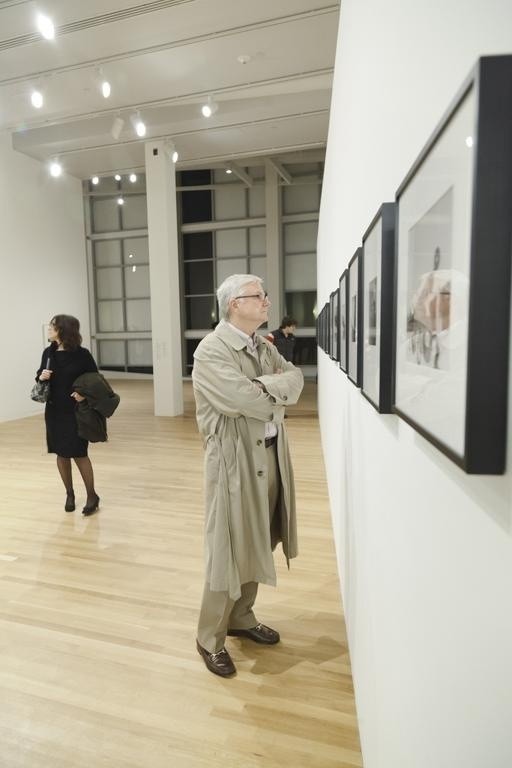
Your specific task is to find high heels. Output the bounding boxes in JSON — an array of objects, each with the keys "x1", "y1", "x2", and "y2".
[
  {"x1": 65, "y1": 494, "x2": 76, "y2": 512},
  {"x1": 82, "y1": 493, "x2": 100, "y2": 514}
]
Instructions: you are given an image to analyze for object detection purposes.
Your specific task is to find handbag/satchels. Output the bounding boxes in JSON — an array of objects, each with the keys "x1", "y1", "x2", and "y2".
[{"x1": 31, "y1": 380, "x2": 51, "y2": 403}]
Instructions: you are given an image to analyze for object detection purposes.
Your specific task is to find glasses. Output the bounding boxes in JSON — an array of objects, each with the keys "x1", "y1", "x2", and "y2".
[
  {"x1": 47, "y1": 322, "x2": 55, "y2": 327},
  {"x1": 235, "y1": 292, "x2": 268, "y2": 301}
]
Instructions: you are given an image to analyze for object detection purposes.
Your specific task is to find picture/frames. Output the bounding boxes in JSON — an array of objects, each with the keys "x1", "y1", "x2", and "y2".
[
  {"x1": 329, "y1": 245, "x2": 360, "y2": 390},
  {"x1": 361, "y1": 202, "x2": 395, "y2": 414},
  {"x1": 393, "y1": 55, "x2": 511, "y2": 476}
]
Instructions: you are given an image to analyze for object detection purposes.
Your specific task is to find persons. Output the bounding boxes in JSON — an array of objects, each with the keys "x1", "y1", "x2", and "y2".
[
  {"x1": 191, "y1": 273, "x2": 306, "y2": 676},
  {"x1": 35, "y1": 314, "x2": 99, "y2": 514},
  {"x1": 273, "y1": 317, "x2": 298, "y2": 418},
  {"x1": 401, "y1": 268, "x2": 456, "y2": 367}
]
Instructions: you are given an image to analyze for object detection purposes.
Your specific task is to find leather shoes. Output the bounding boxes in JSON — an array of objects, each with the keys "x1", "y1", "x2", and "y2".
[
  {"x1": 226, "y1": 623, "x2": 280, "y2": 645},
  {"x1": 196, "y1": 638, "x2": 237, "y2": 678}
]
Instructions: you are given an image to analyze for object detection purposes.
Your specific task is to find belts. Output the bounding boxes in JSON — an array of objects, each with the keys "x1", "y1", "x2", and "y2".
[{"x1": 265, "y1": 436, "x2": 277, "y2": 448}]
